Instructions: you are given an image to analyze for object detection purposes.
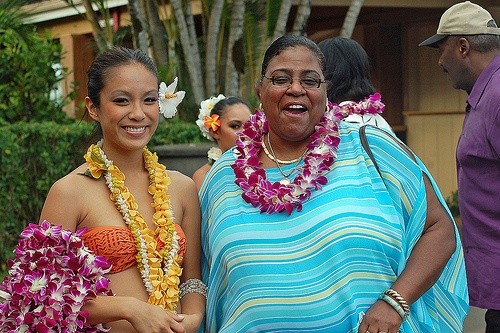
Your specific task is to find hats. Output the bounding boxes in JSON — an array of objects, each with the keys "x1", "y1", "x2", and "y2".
[{"x1": 418, "y1": 1, "x2": 500, "y2": 48}]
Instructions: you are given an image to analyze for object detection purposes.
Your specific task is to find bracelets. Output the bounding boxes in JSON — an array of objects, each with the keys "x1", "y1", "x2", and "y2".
[
  {"x1": 377, "y1": 295, "x2": 407, "y2": 322},
  {"x1": 180, "y1": 279, "x2": 208, "y2": 301},
  {"x1": 384, "y1": 289, "x2": 409, "y2": 318}
]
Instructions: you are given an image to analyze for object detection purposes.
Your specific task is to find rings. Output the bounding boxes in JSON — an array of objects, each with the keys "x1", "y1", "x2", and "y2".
[{"x1": 366, "y1": 331, "x2": 370, "y2": 333}]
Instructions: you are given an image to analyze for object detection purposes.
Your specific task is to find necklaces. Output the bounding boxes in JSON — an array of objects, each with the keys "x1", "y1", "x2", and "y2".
[
  {"x1": 231, "y1": 97, "x2": 344, "y2": 215},
  {"x1": 208, "y1": 147, "x2": 222, "y2": 167},
  {"x1": 77, "y1": 138, "x2": 183, "y2": 316},
  {"x1": 341, "y1": 93, "x2": 385, "y2": 117}
]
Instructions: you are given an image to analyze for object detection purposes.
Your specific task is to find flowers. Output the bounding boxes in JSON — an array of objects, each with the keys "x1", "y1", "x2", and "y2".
[
  {"x1": 158, "y1": 76, "x2": 186, "y2": 119},
  {"x1": 207, "y1": 146, "x2": 223, "y2": 166},
  {"x1": 203, "y1": 113, "x2": 222, "y2": 133},
  {"x1": 195, "y1": 93, "x2": 226, "y2": 142}
]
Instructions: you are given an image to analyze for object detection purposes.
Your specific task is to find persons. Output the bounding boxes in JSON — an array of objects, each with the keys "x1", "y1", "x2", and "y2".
[
  {"x1": 418, "y1": 1, "x2": 500, "y2": 333},
  {"x1": 197, "y1": 33, "x2": 469, "y2": 333},
  {"x1": 38, "y1": 46, "x2": 205, "y2": 333},
  {"x1": 192, "y1": 94, "x2": 253, "y2": 191},
  {"x1": 318, "y1": 37, "x2": 396, "y2": 135}
]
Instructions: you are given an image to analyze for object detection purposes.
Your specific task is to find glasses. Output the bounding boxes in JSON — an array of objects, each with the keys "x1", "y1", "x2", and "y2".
[{"x1": 264, "y1": 75, "x2": 327, "y2": 89}]
